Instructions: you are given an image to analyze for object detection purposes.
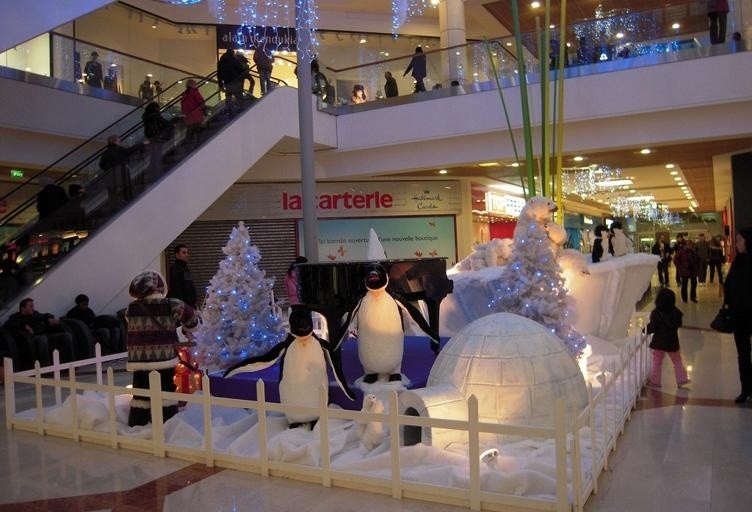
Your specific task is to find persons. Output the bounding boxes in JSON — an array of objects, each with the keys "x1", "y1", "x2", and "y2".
[
  {"x1": 636, "y1": 213, "x2": 751, "y2": 409},
  {"x1": 170, "y1": 241, "x2": 200, "y2": 317},
  {"x1": 9, "y1": 288, "x2": 65, "y2": 377},
  {"x1": 121, "y1": 270, "x2": 203, "y2": 426},
  {"x1": 283, "y1": 252, "x2": 311, "y2": 306},
  {"x1": 99, "y1": 135, "x2": 157, "y2": 213},
  {"x1": 178, "y1": 78, "x2": 209, "y2": 150},
  {"x1": 64, "y1": 289, "x2": 123, "y2": 356},
  {"x1": 142, "y1": 102, "x2": 186, "y2": 181},
  {"x1": 33, "y1": 173, "x2": 88, "y2": 261}
]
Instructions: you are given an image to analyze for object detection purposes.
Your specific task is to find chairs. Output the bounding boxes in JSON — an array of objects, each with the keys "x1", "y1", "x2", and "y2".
[{"x1": 1, "y1": 314, "x2": 127, "y2": 375}]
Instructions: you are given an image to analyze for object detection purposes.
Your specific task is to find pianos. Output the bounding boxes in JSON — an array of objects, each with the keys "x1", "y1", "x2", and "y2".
[{"x1": 289, "y1": 257, "x2": 453, "y2": 372}]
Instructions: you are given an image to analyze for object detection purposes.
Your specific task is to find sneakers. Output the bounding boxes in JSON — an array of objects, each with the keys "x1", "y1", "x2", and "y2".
[
  {"x1": 690, "y1": 298, "x2": 698, "y2": 303},
  {"x1": 645, "y1": 381, "x2": 662, "y2": 390},
  {"x1": 682, "y1": 298, "x2": 688, "y2": 304},
  {"x1": 677, "y1": 379, "x2": 692, "y2": 388}
]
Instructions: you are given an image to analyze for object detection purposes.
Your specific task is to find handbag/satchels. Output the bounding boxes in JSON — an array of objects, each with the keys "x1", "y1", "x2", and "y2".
[{"x1": 710, "y1": 305, "x2": 737, "y2": 335}]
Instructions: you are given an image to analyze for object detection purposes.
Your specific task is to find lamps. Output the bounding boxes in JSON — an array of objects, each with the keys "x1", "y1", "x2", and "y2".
[{"x1": 103, "y1": 0, "x2": 441, "y2": 50}]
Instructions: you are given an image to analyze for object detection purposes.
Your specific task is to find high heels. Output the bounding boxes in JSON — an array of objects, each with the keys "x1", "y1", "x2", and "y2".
[{"x1": 735, "y1": 388, "x2": 752, "y2": 404}]
